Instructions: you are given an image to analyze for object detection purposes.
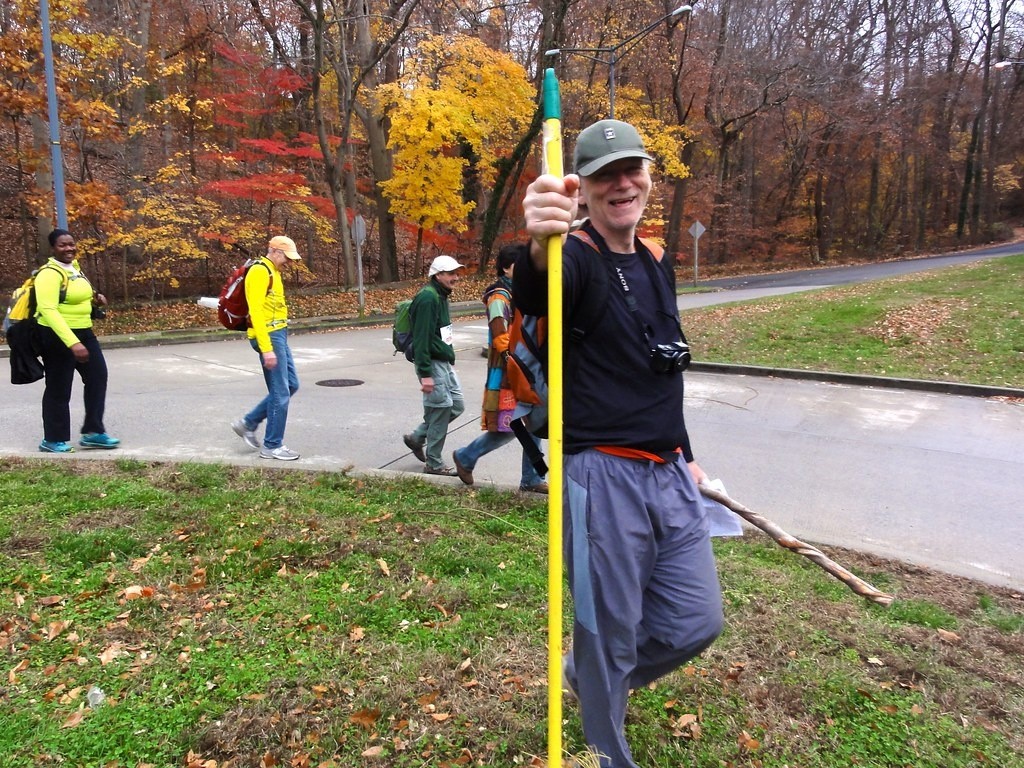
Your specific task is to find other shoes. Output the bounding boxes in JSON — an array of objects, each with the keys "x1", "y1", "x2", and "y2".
[
  {"x1": 425, "y1": 460, "x2": 458, "y2": 476},
  {"x1": 403, "y1": 434, "x2": 426, "y2": 463},
  {"x1": 452, "y1": 449, "x2": 473, "y2": 484},
  {"x1": 520, "y1": 481, "x2": 549, "y2": 493}
]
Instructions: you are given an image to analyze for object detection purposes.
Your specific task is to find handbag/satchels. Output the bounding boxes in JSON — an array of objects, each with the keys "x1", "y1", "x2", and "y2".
[{"x1": 9, "y1": 355, "x2": 44, "y2": 384}]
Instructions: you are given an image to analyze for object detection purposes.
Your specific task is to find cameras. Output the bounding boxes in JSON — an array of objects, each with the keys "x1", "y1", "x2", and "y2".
[
  {"x1": 649, "y1": 342, "x2": 692, "y2": 375},
  {"x1": 91, "y1": 306, "x2": 106, "y2": 320}
]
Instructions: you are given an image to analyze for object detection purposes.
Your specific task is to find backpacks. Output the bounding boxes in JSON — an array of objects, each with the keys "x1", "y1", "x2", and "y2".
[
  {"x1": 392, "y1": 286, "x2": 441, "y2": 363},
  {"x1": 217, "y1": 259, "x2": 273, "y2": 332},
  {"x1": 1, "y1": 264, "x2": 68, "y2": 354},
  {"x1": 506, "y1": 217, "x2": 677, "y2": 440}
]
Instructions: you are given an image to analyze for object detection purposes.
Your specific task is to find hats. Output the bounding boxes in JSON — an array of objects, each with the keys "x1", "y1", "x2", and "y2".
[
  {"x1": 269, "y1": 236, "x2": 303, "y2": 259},
  {"x1": 428, "y1": 255, "x2": 465, "y2": 277},
  {"x1": 572, "y1": 120, "x2": 653, "y2": 177}
]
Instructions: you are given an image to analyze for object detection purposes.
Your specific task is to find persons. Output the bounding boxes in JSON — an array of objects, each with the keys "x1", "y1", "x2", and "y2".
[
  {"x1": 402, "y1": 255, "x2": 465, "y2": 476},
  {"x1": 512, "y1": 120, "x2": 724, "y2": 768},
  {"x1": 230, "y1": 236, "x2": 301, "y2": 460},
  {"x1": 453, "y1": 243, "x2": 548, "y2": 494},
  {"x1": 32, "y1": 230, "x2": 120, "y2": 453}
]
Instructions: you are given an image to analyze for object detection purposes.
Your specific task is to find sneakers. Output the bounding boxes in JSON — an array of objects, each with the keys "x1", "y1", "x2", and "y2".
[
  {"x1": 38, "y1": 439, "x2": 75, "y2": 454},
  {"x1": 79, "y1": 432, "x2": 120, "y2": 449},
  {"x1": 259, "y1": 445, "x2": 300, "y2": 460},
  {"x1": 230, "y1": 420, "x2": 260, "y2": 448}
]
